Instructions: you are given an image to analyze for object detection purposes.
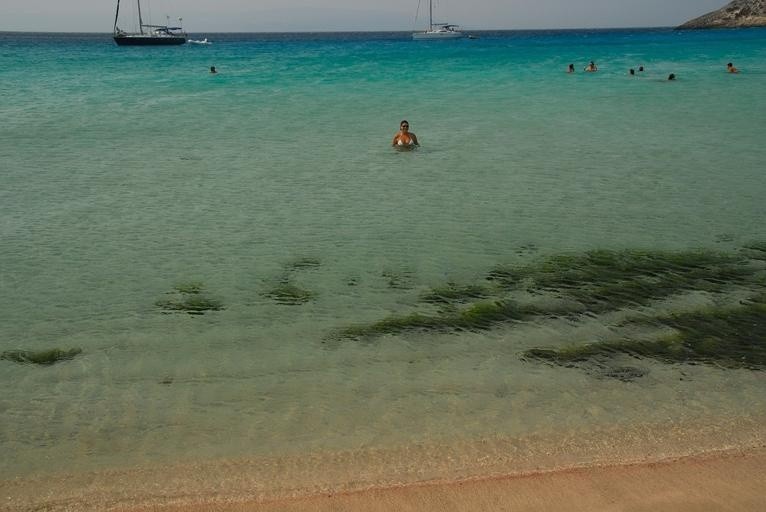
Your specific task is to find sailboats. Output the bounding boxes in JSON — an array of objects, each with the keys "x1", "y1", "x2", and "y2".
[
  {"x1": 411, "y1": 1, "x2": 464, "y2": 41},
  {"x1": 113, "y1": 0, "x2": 186, "y2": 46}
]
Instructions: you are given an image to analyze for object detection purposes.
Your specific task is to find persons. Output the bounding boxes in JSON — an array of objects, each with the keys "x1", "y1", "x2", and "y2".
[
  {"x1": 727, "y1": 62, "x2": 737, "y2": 73},
  {"x1": 564, "y1": 61, "x2": 678, "y2": 83},
  {"x1": 392, "y1": 120, "x2": 418, "y2": 147},
  {"x1": 209, "y1": 67, "x2": 217, "y2": 73}
]
